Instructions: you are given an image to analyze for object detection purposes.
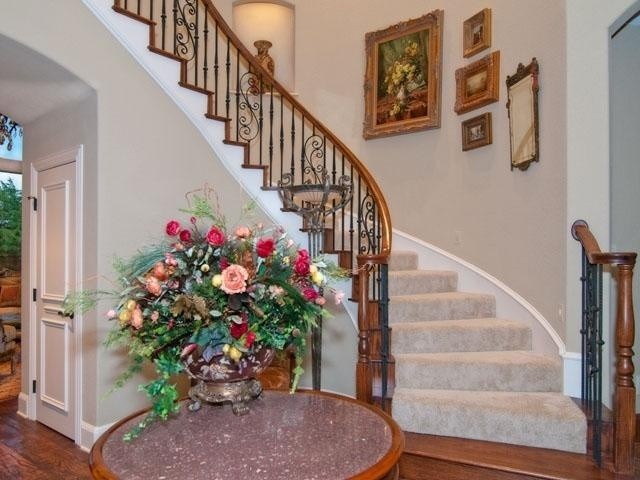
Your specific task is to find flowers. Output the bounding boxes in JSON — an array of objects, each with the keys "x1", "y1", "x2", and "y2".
[{"x1": 59, "y1": 181, "x2": 349, "y2": 441}]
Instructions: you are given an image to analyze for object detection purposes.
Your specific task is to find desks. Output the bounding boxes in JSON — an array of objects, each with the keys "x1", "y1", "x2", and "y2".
[
  {"x1": 0, "y1": 312, "x2": 22, "y2": 367},
  {"x1": 86, "y1": 394, "x2": 408, "y2": 480}
]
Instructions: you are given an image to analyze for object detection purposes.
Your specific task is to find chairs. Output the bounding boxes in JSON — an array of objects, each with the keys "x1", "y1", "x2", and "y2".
[{"x1": 1, "y1": 318, "x2": 18, "y2": 376}]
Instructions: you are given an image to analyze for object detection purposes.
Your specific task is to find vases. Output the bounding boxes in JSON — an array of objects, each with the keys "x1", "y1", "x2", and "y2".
[{"x1": 173, "y1": 337, "x2": 270, "y2": 420}]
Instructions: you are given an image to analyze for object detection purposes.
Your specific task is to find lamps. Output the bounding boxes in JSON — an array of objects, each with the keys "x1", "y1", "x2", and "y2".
[{"x1": 229, "y1": 0, "x2": 297, "y2": 99}]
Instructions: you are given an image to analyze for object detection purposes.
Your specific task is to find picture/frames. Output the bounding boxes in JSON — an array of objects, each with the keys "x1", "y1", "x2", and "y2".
[
  {"x1": 463, "y1": 6, "x2": 493, "y2": 58},
  {"x1": 459, "y1": 112, "x2": 494, "y2": 150},
  {"x1": 364, "y1": 7, "x2": 447, "y2": 139},
  {"x1": 452, "y1": 52, "x2": 503, "y2": 114},
  {"x1": 505, "y1": 56, "x2": 541, "y2": 171}
]
